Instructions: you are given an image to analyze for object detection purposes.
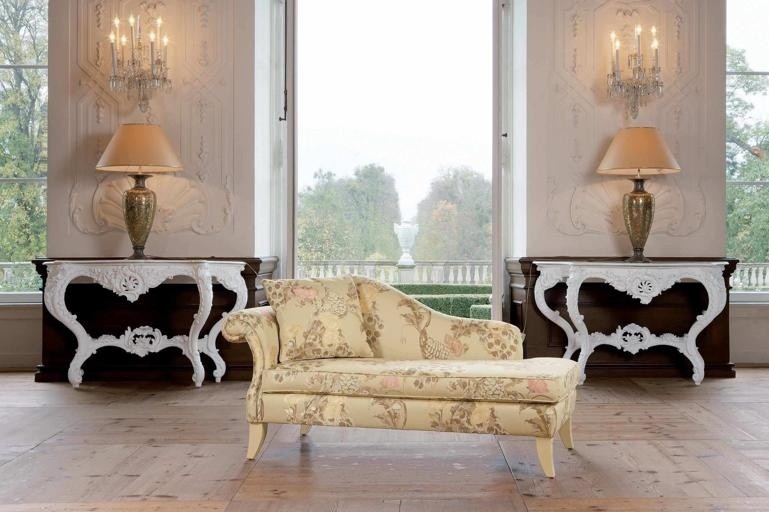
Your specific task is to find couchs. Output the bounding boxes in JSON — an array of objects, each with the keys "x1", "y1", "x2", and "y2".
[{"x1": 223, "y1": 276, "x2": 582, "y2": 480}]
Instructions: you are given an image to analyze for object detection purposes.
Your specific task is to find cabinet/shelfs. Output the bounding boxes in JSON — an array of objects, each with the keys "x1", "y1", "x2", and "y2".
[
  {"x1": 504, "y1": 256, "x2": 739, "y2": 378},
  {"x1": 32, "y1": 256, "x2": 280, "y2": 383}
]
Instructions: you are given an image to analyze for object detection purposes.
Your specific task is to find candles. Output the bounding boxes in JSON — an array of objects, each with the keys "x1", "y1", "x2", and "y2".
[
  {"x1": 606, "y1": 25, "x2": 664, "y2": 79},
  {"x1": 106, "y1": 13, "x2": 171, "y2": 73}
]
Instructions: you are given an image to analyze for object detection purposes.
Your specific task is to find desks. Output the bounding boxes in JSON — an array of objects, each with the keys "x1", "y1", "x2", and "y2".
[
  {"x1": 43, "y1": 258, "x2": 249, "y2": 389},
  {"x1": 532, "y1": 261, "x2": 730, "y2": 385}
]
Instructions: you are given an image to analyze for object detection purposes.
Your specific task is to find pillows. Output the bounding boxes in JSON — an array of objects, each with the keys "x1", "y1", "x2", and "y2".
[{"x1": 264, "y1": 276, "x2": 376, "y2": 363}]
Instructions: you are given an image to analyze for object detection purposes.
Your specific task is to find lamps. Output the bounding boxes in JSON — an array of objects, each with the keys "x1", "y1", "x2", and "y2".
[
  {"x1": 597, "y1": 128, "x2": 682, "y2": 264},
  {"x1": 95, "y1": 122, "x2": 183, "y2": 260}
]
